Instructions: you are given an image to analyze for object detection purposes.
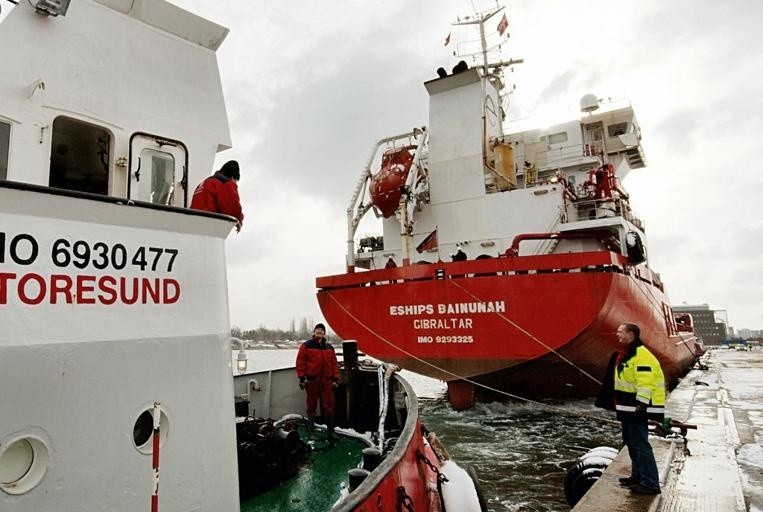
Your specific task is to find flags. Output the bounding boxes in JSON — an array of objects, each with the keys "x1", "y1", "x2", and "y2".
[{"x1": 496, "y1": 13, "x2": 508, "y2": 35}]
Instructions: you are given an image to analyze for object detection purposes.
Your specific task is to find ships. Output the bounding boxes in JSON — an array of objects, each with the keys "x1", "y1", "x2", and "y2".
[
  {"x1": 316, "y1": 0, "x2": 698, "y2": 412},
  {"x1": 0, "y1": 0, "x2": 489, "y2": 512}
]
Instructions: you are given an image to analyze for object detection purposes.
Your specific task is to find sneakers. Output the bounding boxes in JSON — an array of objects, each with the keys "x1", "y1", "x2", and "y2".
[
  {"x1": 618, "y1": 475, "x2": 661, "y2": 494},
  {"x1": 305, "y1": 421, "x2": 341, "y2": 443}
]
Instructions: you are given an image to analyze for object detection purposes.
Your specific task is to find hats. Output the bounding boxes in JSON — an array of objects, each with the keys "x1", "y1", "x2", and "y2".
[
  {"x1": 219, "y1": 159, "x2": 242, "y2": 181},
  {"x1": 313, "y1": 323, "x2": 326, "y2": 335}
]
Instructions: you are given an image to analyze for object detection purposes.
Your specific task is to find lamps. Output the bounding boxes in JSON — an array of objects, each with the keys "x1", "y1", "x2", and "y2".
[{"x1": 34, "y1": 0, "x2": 71, "y2": 17}]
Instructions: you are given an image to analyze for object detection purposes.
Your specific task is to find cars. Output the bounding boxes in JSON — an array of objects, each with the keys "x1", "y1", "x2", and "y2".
[
  {"x1": 728, "y1": 343, "x2": 734, "y2": 348},
  {"x1": 722, "y1": 344, "x2": 729, "y2": 349},
  {"x1": 735, "y1": 344, "x2": 747, "y2": 351}
]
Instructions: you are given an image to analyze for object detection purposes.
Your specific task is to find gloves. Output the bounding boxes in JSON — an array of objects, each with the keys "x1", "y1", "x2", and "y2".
[
  {"x1": 298, "y1": 376, "x2": 308, "y2": 392},
  {"x1": 330, "y1": 375, "x2": 339, "y2": 389}
]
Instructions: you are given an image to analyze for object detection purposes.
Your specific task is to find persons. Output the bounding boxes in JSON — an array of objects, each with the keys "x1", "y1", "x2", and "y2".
[
  {"x1": 455, "y1": 247, "x2": 468, "y2": 278},
  {"x1": 189, "y1": 160, "x2": 243, "y2": 233},
  {"x1": 296, "y1": 323, "x2": 340, "y2": 441},
  {"x1": 611, "y1": 320, "x2": 666, "y2": 494},
  {"x1": 384, "y1": 255, "x2": 398, "y2": 284}
]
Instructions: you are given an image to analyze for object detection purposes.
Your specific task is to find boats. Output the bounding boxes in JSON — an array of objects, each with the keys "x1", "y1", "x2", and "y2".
[{"x1": 694, "y1": 329, "x2": 707, "y2": 356}]
